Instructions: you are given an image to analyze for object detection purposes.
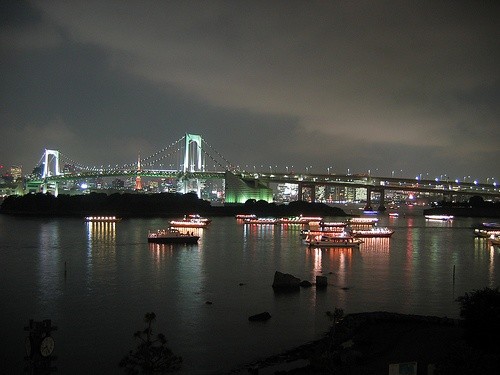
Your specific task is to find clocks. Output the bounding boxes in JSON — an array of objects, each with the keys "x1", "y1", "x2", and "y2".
[
  {"x1": 24, "y1": 334, "x2": 34, "y2": 359},
  {"x1": 39, "y1": 336, "x2": 55, "y2": 358}
]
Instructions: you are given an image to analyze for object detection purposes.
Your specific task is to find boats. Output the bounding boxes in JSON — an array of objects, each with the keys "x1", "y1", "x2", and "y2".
[
  {"x1": 279, "y1": 215, "x2": 395, "y2": 248},
  {"x1": 384, "y1": 200, "x2": 432, "y2": 210},
  {"x1": 83, "y1": 217, "x2": 123, "y2": 222},
  {"x1": 146, "y1": 228, "x2": 200, "y2": 242},
  {"x1": 474, "y1": 221, "x2": 500, "y2": 244},
  {"x1": 424, "y1": 215, "x2": 455, "y2": 220},
  {"x1": 168, "y1": 214, "x2": 213, "y2": 228},
  {"x1": 237, "y1": 214, "x2": 278, "y2": 223}
]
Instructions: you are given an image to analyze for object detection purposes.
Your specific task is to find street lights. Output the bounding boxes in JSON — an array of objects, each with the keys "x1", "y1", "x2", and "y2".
[
  {"x1": 85, "y1": 163, "x2": 126, "y2": 175},
  {"x1": 141, "y1": 162, "x2": 241, "y2": 172},
  {"x1": 245, "y1": 164, "x2": 385, "y2": 182},
  {"x1": 391, "y1": 169, "x2": 496, "y2": 190}
]
